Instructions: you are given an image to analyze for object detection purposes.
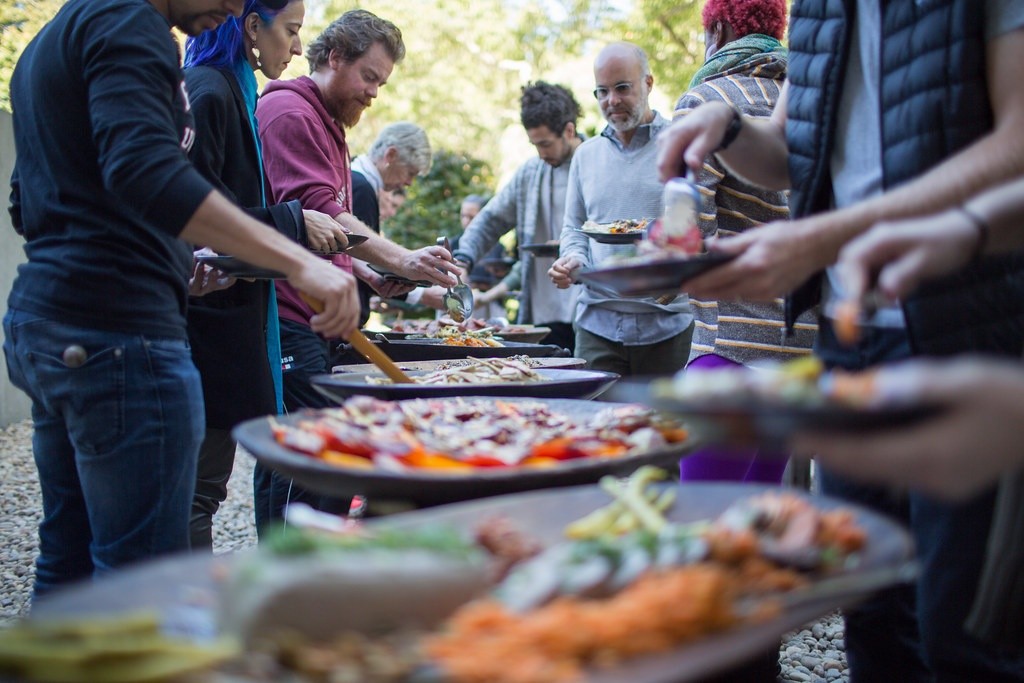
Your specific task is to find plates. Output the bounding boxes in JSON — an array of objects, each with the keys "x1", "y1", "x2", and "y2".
[
  {"x1": 233, "y1": 398, "x2": 708, "y2": 494},
  {"x1": 518, "y1": 241, "x2": 562, "y2": 260},
  {"x1": 568, "y1": 254, "x2": 733, "y2": 297},
  {"x1": 195, "y1": 255, "x2": 291, "y2": 281},
  {"x1": 570, "y1": 218, "x2": 656, "y2": 245},
  {"x1": 361, "y1": 317, "x2": 552, "y2": 342},
  {"x1": 317, "y1": 232, "x2": 369, "y2": 254},
  {"x1": 479, "y1": 256, "x2": 515, "y2": 268},
  {"x1": 370, "y1": 338, "x2": 561, "y2": 362},
  {"x1": 33, "y1": 482, "x2": 914, "y2": 683},
  {"x1": 311, "y1": 355, "x2": 621, "y2": 399},
  {"x1": 709, "y1": 485, "x2": 714, "y2": 513},
  {"x1": 365, "y1": 262, "x2": 446, "y2": 288}
]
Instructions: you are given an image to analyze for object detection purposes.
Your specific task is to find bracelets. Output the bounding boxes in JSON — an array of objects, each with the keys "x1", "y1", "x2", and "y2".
[
  {"x1": 954, "y1": 200, "x2": 987, "y2": 270},
  {"x1": 707, "y1": 108, "x2": 741, "y2": 154}
]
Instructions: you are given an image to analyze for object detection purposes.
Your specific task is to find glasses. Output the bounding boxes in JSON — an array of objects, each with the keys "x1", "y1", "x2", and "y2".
[{"x1": 593, "y1": 75, "x2": 647, "y2": 100}]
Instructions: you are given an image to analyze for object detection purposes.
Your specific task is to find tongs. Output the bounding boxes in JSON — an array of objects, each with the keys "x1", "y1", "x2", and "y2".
[
  {"x1": 435, "y1": 233, "x2": 473, "y2": 324},
  {"x1": 659, "y1": 154, "x2": 707, "y2": 242}
]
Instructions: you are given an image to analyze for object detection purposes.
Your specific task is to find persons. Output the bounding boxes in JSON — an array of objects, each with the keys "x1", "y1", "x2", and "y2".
[
  {"x1": 349, "y1": 123, "x2": 448, "y2": 330},
  {"x1": 657, "y1": 0, "x2": 1024, "y2": 683},
  {"x1": 2, "y1": 0, "x2": 362, "y2": 623},
  {"x1": 668, "y1": 0, "x2": 818, "y2": 486},
  {"x1": 443, "y1": 78, "x2": 592, "y2": 358},
  {"x1": 427, "y1": 191, "x2": 514, "y2": 325},
  {"x1": 255, "y1": 8, "x2": 464, "y2": 542},
  {"x1": 545, "y1": 39, "x2": 694, "y2": 383},
  {"x1": 180, "y1": 0, "x2": 348, "y2": 553}
]
